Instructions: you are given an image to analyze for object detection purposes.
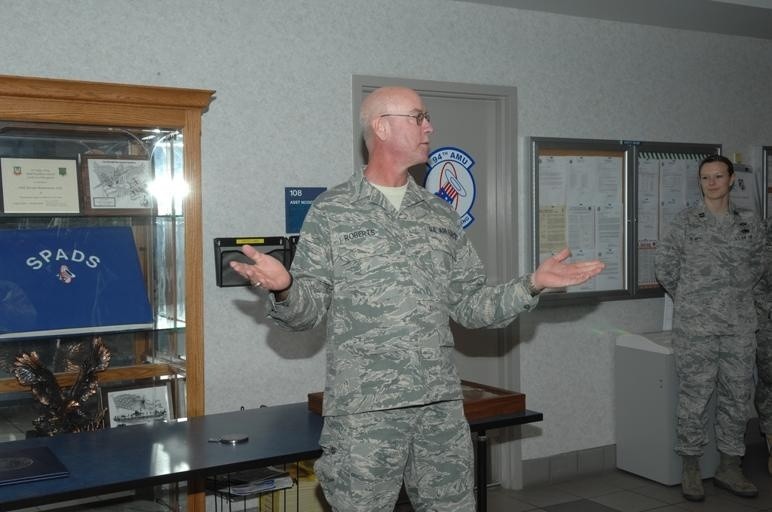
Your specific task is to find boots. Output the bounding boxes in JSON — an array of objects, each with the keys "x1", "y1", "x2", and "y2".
[
  {"x1": 680, "y1": 453, "x2": 706, "y2": 503},
  {"x1": 712, "y1": 449, "x2": 760, "y2": 498}
]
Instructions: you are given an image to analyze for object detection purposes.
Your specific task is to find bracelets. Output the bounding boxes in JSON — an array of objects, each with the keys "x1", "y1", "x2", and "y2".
[
  {"x1": 527, "y1": 277, "x2": 541, "y2": 297},
  {"x1": 267, "y1": 273, "x2": 294, "y2": 292}
]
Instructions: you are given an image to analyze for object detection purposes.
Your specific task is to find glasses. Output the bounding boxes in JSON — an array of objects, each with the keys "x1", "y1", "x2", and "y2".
[{"x1": 380, "y1": 111, "x2": 432, "y2": 127}]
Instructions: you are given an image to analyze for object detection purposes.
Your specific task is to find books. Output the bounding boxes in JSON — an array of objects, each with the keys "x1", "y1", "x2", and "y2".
[
  {"x1": 209, "y1": 466, "x2": 296, "y2": 498},
  {"x1": 0, "y1": 446, "x2": 70, "y2": 485}
]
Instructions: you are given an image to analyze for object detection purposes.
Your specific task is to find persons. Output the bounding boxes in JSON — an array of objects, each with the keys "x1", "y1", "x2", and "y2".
[
  {"x1": 654, "y1": 155, "x2": 765, "y2": 500},
  {"x1": 229, "y1": 86, "x2": 607, "y2": 512},
  {"x1": 752, "y1": 216, "x2": 771, "y2": 479}
]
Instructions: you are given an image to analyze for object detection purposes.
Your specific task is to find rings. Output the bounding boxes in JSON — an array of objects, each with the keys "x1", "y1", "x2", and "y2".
[{"x1": 254, "y1": 282, "x2": 261, "y2": 286}]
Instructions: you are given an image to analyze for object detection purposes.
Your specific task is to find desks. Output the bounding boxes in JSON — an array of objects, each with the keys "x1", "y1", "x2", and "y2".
[{"x1": 1, "y1": 387, "x2": 544, "y2": 511}]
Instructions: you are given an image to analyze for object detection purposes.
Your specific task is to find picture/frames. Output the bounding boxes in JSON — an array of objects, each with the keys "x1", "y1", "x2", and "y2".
[{"x1": 76, "y1": 150, "x2": 158, "y2": 218}]
